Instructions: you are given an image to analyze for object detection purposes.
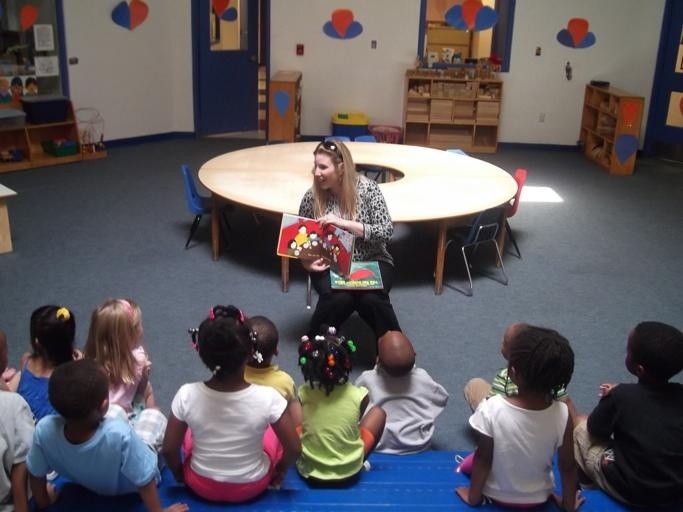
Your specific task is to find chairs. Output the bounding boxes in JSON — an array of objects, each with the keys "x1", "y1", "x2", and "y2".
[
  {"x1": 500, "y1": 166, "x2": 527, "y2": 257},
  {"x1": 323, "y1": 134, "x2": 388, "y2": 184},
  {"x1": 434, "y1": 205, "x2": 511, "y2": 296},
  {"x1": 182, "y1": 165, "x2": 234, "y2": 248}
]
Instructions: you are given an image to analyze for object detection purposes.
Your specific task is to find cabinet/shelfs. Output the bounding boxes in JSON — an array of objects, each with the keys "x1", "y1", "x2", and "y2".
[
  {"x1": 578, "y1": 80, "x2": 644, "y2": 175},
  {"x1": 404, "y1": 69, "x2": 503, "y2": 155},
  {"x1": 268, "y1": 70, "x2": 302, "y2": 141},
  {"x1": 2, "y1": 100, "x2": 107, "y2": 171}
]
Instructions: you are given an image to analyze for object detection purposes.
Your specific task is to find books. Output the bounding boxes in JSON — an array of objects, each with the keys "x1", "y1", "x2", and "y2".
[
  {"x1": 275, "y1": 211, "x2": 357, "y2": 283},
  {"x1": 328, "y1": 259, "x2": 385, "y2": 291}
]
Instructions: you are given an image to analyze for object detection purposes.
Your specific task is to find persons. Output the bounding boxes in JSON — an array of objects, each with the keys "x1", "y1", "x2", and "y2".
[
  {"x1": 573, "y1": 321, "x2": 683, "y2": 511},
  {"x1": 354, "y1": 331, "x2": 450, "y2": 456},
  {"x1": 464, "y1": 321, "x2": 578, "y2": 430},
  {"x1": 243, "y1": 316, "x2": 301, "y2": 425},
  {"x1": 164, "y1": 304, "x2": 301, "y2": 504},
  {"x1": 296, "y1": 324, "x2": 387, "y2": 487},
  {"x1": 454, "y1": 328, "x2": 588, "y2": 512},
  {"x1": 299, "y1": 139, "x2": 402, "y2": 359},
  {"x1": 81, "y1": 298, "x2": 160, "y2": 425},
  {"x1": 0, "y1": 328, "x2": 35, "y2": 512},
  {"x1": 17, "y1": 305, "x2": 83, "y2": 418},
  {"x1": 27, "y1": 359, "x2": 187, "y2": 512}
]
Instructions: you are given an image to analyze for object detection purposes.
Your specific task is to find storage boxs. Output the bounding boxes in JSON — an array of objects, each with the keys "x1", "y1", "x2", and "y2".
[
  {"x1": 23, "y1": 94, "x2": 68, "y2": 123},
  {"x1": 332, "y1": 113, "x2": 370, "y2": 138}
]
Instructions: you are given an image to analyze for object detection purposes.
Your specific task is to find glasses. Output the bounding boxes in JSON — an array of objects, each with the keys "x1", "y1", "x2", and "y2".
[{"x1": 319, "y1": 140, "x2": 343, "y2": 163}]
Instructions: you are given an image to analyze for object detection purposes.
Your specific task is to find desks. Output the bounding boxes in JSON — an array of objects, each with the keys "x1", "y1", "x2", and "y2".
[{"x1": 199, "y1": 142, "x2": 517, "y2": 308}]
